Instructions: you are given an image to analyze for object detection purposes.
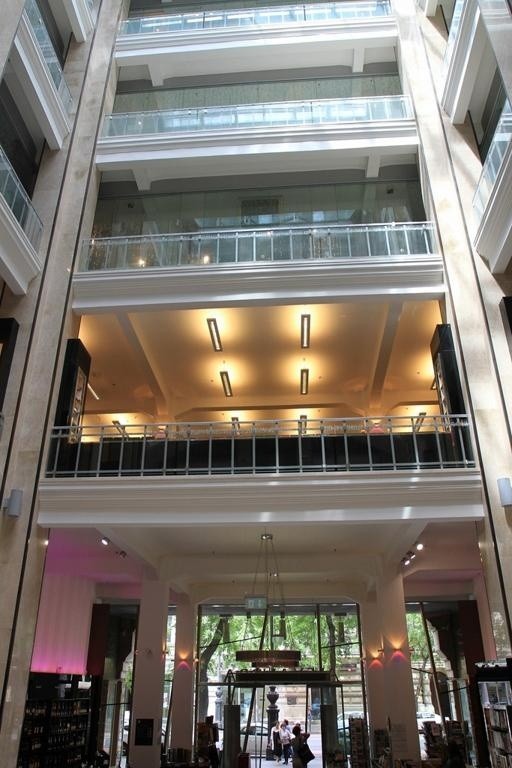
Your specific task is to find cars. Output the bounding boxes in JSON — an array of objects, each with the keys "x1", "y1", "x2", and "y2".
[
  {"x1": 120, "y1": 723, "x2": 171, "y2": 757},
  {"x1": 308, "y1": 702, "x2": 326, "y2": 719},
  {"x1": 337, "y1": 712, "x2": 370, "y2": 741},
  {"x1": 416, "y1": 712, "x2": 450, "y2": 735},
  {"x1": 218, "y1": 724, "x2": 295, "y2": 757}
]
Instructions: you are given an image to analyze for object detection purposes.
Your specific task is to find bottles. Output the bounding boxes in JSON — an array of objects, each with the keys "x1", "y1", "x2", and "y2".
[{"x1": 17, "y1": 699, "x2": 87, "y2": 768}]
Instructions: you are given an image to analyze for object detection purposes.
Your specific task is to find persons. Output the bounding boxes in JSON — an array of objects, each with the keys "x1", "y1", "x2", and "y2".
[
  {"x1": 269, "y1": 721, "x2": 283, "y2": 765},
  {"x1": 289, "y1": 725, "x2": 311, "y2": 768},
  {"x1": 280, "y1": 724, "x2": 292, "y2": 765},
  {"x1": 282, "y1": 719, "x2": 292, "y2": 758},
  {"x1": 295, "y1": 722, "x2": 304, "y2": 732}
]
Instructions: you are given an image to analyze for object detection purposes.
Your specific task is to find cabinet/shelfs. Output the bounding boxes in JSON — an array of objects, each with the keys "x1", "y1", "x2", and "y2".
[
  {"x1": 18, "y1": 671, "x2": 105, "y2": 768},
  {"x1": 465, "y1": 657, "x2": 511, "y2": 768}
]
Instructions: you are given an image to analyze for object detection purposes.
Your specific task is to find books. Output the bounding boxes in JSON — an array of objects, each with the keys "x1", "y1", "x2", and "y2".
[{"x1": 349, "y1": 708, "x2": 511, "y2": 768}]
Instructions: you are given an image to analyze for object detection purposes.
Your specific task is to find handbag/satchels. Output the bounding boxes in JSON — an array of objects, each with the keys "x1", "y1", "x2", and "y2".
[{"x1": 297, "y1": 744, "x2": 315, "y2": 764}]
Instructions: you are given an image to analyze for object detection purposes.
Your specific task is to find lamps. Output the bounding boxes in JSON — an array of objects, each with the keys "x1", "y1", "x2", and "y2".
[
  {"x1": 2, "y1": 489, "x2": 23, "y2": 518},
  {"x1": 98, "y1": 537, "x2": 128, "y2": 561},
  {"x1": 497, "y1": 477, "x2": 512, "y2": 507},
  {"x1": 402, "y1": 542, "x2": 424, "y2": 566}
]
